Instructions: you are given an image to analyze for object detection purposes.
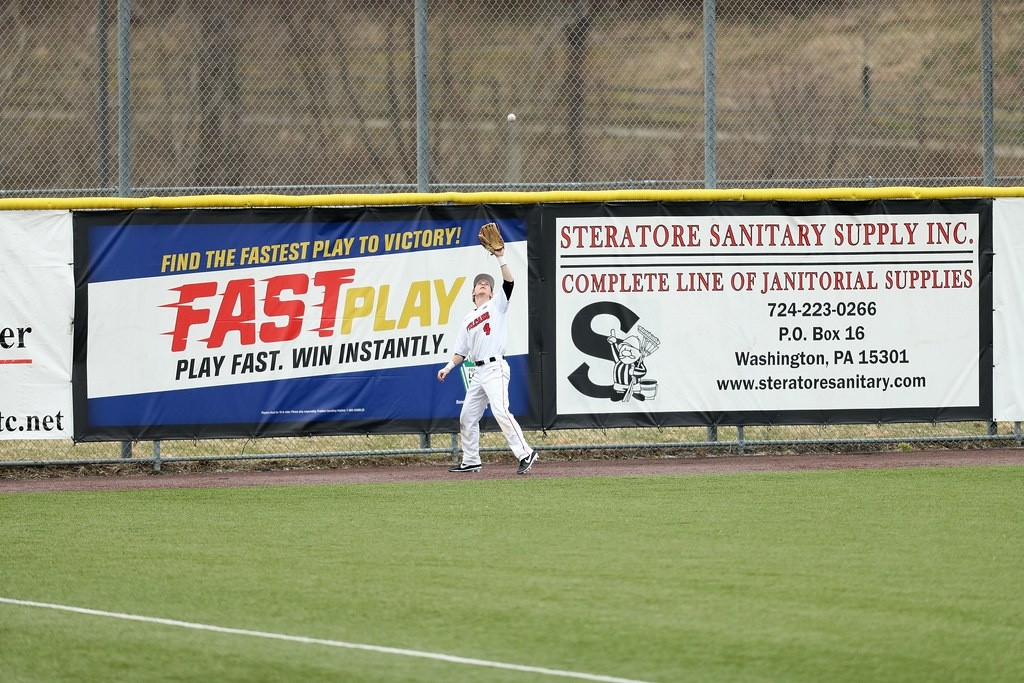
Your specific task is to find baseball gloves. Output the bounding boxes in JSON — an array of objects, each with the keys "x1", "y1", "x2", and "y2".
[{"x1": 478, "y1": 222, "x2": 506, "y2": 256}]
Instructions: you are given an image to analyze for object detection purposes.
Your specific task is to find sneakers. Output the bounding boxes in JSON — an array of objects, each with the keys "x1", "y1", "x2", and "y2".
[
  {"x1": 448, "y1": 460, "x2": 482, "y2": 473},
  {"x1": 517, "y1": 450, "x2": 539, "y2": 474}
]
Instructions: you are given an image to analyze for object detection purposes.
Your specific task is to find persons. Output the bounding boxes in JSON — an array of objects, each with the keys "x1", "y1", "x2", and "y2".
[{"x1": 436, "y1": 247, "x2": 539, "y2": 473}]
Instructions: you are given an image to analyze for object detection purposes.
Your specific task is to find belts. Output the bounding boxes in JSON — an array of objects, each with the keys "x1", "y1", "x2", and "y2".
[{"x1": 475, "y1": 356, "x2": 504, "y2": 366}]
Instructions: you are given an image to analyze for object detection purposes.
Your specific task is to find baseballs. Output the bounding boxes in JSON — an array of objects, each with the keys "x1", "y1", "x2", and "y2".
[{"x1": 508, "y1": 113, "x2": 516, "y2": 123}]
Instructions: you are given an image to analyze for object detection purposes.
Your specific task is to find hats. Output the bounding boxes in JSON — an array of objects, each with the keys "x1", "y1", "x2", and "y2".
[{"x1": 473, "y1": 274, "x2": 495, "y2": 290}]
